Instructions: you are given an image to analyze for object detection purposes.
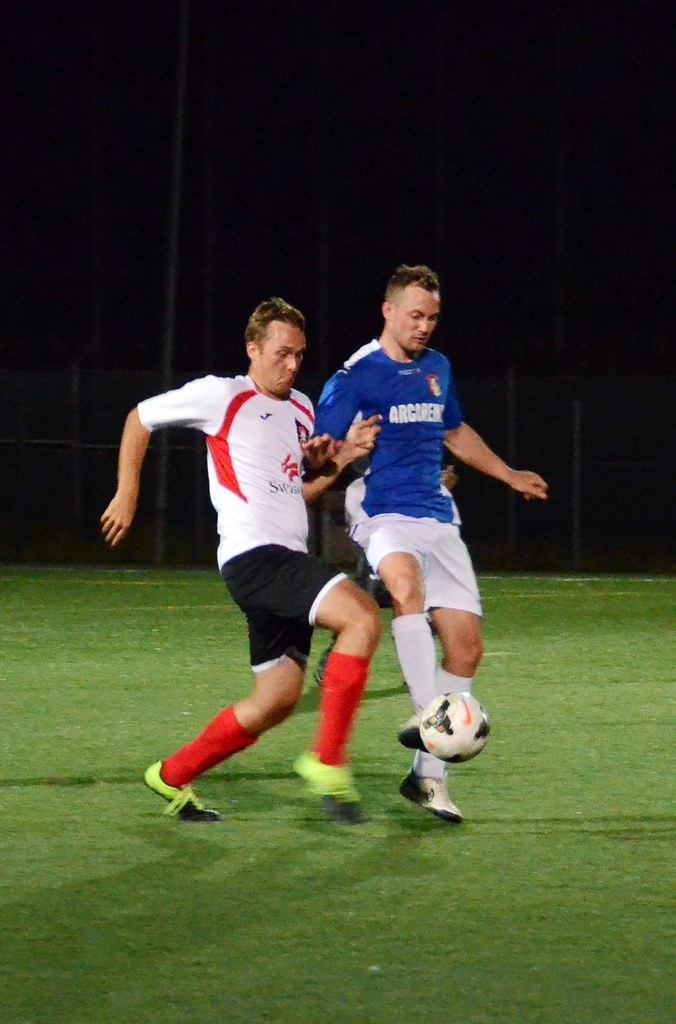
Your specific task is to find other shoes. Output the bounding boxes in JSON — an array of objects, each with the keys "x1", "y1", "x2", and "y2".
[{"x1": 314, "y1": 652, "x2": 329, "y2": 684}]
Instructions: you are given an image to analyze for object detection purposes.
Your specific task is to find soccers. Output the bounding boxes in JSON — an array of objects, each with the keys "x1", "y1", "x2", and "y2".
[{"x1": 420, "y1": 691, "x2": 489, "y2": 764}]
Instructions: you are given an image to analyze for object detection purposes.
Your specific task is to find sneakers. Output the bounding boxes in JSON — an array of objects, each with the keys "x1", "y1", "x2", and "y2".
[
  {"x1": 399, "y1": 766, "x2": 463, "y2": 823},
  {"x1": 400, "y1": 719, "x2": 432, "y2": 753},
  {"x1": 143, "y1": 760, "x2": 221, "y2": 822},
  {"x1": 293, "y1": 748, "x2": 361, "y2": 814}
]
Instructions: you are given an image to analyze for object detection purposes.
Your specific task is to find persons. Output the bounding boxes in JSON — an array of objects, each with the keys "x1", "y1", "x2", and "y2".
[
  {"x1": 312, "y1": 265, "x2": 548, "y2": 825},
  {"x1": 98, "y1": 296, "x2": 382, "y2": 823}
]
